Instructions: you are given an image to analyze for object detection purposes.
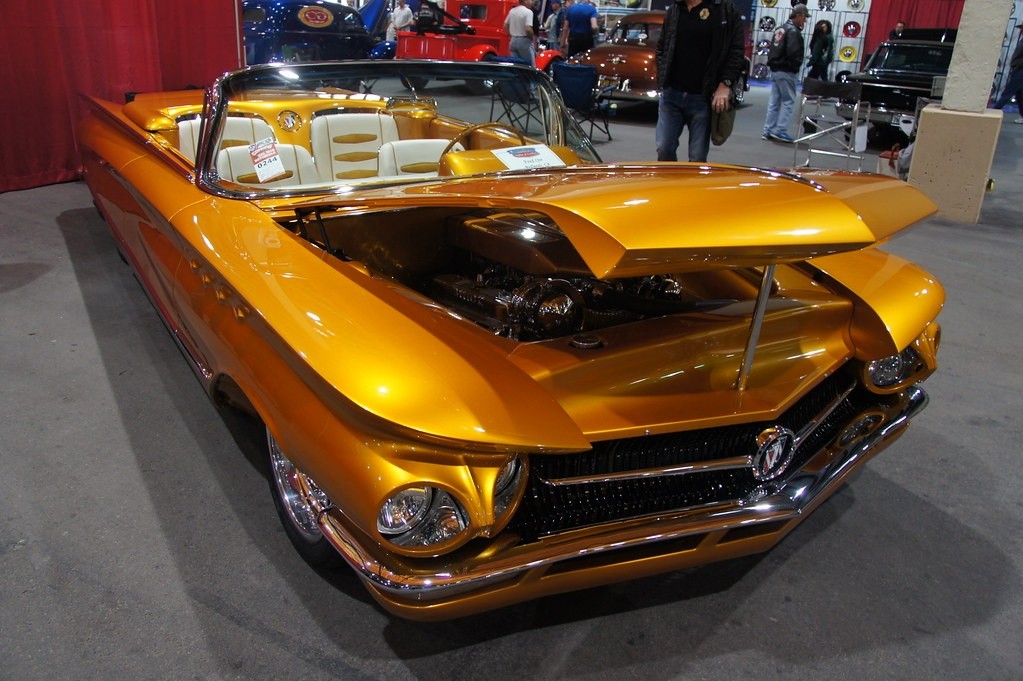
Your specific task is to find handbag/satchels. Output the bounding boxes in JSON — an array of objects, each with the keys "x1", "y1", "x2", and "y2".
[{"x1": 711, "y1": 107, "x2": 736, "y2": 145}]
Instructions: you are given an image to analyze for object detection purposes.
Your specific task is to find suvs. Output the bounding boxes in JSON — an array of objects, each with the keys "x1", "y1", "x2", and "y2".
[{"x1": 844, "y1": 38, "x2": 954, "y2": 141}]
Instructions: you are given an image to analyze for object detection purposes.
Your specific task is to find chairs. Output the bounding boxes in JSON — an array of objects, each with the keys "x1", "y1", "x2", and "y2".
[
  {"x1": 487, "y1": 56, "x2": 548, "y2": 136},
  {"x1": 176, "y1": 110, "x2": 277, "y2": 167},
  {"x1": 792, "y1": 78, "x2": 872, "y2": 171},
  {"x1": 551, "y1": 61, "x2": 617, "y2": 145},
  {"x1": 216, "y1": 142, "x2": 321, "y2": 188},
  {"x1": 309, "y1": 108, "x2": 399, "y2": 183},
  {"x1": 378, "y1": 138, "x2": 466, "y2": 179}
]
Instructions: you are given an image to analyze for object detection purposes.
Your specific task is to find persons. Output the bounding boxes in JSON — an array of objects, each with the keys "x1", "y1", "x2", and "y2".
[
  {"x1": 529, "y1": 0, "x2": 539, "y2": 50},
  {"x1": 803, "y1": 20, "x2": 834, "y2": 101},
  {"x1": 888, "y1": 21, "x2": 905, "y2": 40},
  {"x1": 394, "y1": 12, "x2": 419, "y2": 32},
  {"x1": 503, "y1": 0, "x2": 535, "y2": 66},
  {"x1": 414, "y1": 3, "x2": 439, "y2": 33},
  {"x1": 654, "y1": 0, "x2": 745, "y2": 162},
  {"x1": 762, "y1": 3, "x2": 812, "y2": 143},
  {"x1": 385, "y1": 0, "x2": 414, "y2": 41},
  {"x1": 544, "y1": 0, "x2": 602, "y2": 60},
  {"x1": 992, "y1": 22, "x2": 1023, "y2": 124}
]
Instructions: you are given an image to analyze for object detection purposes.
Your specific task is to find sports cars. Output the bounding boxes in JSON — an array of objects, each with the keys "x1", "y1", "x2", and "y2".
[{"x1": 77, "y1": 59, "x2": 944, "y2": 620}]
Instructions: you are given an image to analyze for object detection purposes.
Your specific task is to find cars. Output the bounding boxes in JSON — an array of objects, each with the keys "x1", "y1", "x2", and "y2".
[
  {"x1": 566, "y1": 10, "x2": 750, "y2": 109},
  {"x1": 241, "y1": 0, "x2": 395, "y2": 89}
]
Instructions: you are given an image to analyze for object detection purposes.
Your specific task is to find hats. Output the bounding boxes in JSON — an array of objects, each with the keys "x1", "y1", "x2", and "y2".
[
  {"x1": 551, "y1": 0, "x2": 564, "y2": 3},
  {"x1": 792, "y1": 4, "x2": 811, "y2": 18}
]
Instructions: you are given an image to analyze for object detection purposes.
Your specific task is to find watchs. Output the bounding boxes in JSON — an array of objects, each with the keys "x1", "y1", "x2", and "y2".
[{"x1": 722, "y1": 79, "x2": 733, "y2": 89}]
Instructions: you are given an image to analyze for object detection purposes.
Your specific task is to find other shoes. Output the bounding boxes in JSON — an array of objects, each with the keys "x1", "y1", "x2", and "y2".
[
  {"x1": 761, "y1": 133, "x2": 770, "y2": 140},
  {"x1": 771, "y1": 132, "x2": 793, "y2": 142}
]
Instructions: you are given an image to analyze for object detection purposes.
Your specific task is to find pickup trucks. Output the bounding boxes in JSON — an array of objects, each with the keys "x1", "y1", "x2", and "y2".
[{"x1": 397, "y1": 0, "x2": 522, "y2": 93}]
